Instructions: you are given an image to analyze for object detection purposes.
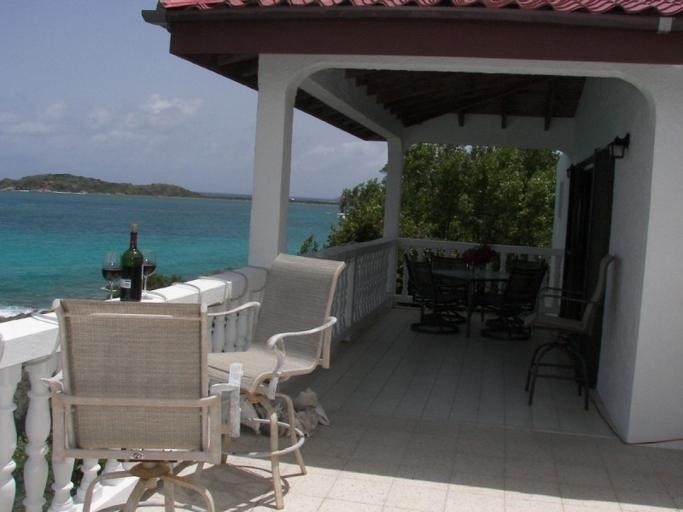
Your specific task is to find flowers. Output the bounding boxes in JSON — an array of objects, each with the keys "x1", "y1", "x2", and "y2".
[{"x1": 463, "y1": 244, "x2": 496, "y2": 266}]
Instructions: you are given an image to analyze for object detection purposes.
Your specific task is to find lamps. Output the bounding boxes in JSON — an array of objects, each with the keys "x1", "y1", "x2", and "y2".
[{"x1": 608, "y1": 133, "x2": 629, "y2": 160}]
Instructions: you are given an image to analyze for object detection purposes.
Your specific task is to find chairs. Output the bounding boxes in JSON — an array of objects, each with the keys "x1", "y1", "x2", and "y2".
[
  {"x1": 404, "y1": 253, "x2": 548, "y2": 341},
  {"x1": 525, "y1": 254, "x2": 615, "y2": 410}
]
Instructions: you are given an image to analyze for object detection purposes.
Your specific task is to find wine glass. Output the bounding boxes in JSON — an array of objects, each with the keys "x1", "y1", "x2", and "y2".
[
  {"x1": 142, "y1": 255, "x2": 157, "y2": 298},
  {"x1": 102, "y1": 252, "x2": 119, "y2": 299}
]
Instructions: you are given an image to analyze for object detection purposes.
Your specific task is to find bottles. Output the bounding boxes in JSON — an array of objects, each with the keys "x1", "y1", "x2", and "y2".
[{"x1": 120, "y1": 223, "x2": 142, "y2": 302}]
